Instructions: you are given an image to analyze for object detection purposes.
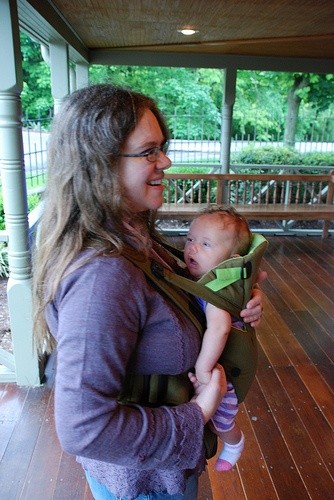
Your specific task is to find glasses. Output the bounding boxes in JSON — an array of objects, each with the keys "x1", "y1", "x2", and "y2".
[{"x1": 119, "y1": 141, "x2": 169, "y2": 162}]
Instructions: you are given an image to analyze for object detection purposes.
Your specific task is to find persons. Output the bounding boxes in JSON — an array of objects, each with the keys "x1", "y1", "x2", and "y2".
[
  {"x1": 183, "y1": 204, "x2": 258, "y2": 472},
  {"x1": 27, "y1": 85, "x2": 264, "y2": 500}
]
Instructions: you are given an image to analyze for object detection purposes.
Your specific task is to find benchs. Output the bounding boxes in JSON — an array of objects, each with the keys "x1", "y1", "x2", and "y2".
[{"x1": 154, "y1": 173, "x2": 334, "y2": 238}]
